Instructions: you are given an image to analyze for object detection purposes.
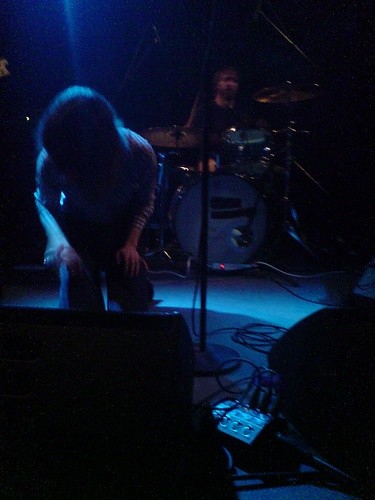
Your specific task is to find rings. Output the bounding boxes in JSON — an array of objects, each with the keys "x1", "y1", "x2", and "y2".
[{"x1": 129, "y1": 260, "x2": 135, "y2": 265}]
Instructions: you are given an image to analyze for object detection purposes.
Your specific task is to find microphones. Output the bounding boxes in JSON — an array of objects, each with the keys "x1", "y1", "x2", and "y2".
[
  {"x1": 236, "y1": 235, "x2": 252, "y2": 248},
  {"x1": 152, "y1": 24, "x2": 162, "y2": 45},
  {"x1": 253, "y1": 0, "x2": 263, "y2": 22}
]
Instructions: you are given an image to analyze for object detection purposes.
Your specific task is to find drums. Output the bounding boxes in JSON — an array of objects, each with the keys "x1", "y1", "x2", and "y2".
[
  {"x1": 167, "y1": 167, "x2": 274, "y2": 273},
  {"x1": 150, "y1": 175, "x2": 174, "y2": 229},
  {"x1": 215, "y1": 123, "x2": 270, "y2": 169}
]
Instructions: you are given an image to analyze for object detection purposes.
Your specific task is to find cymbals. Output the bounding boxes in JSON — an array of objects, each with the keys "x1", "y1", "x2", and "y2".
[
  {"x1": 273, "y1": 129, "x2": 311, "y2": 138},
  {"x1": 141, "y1": 126, "x2": 202, "y2": 148},
  {"x1": 251, "y1": 81, "x2": 324, "y2": 105}
]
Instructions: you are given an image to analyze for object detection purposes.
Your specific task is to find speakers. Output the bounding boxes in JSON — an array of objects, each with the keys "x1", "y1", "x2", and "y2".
[
  {"x1": 267, "y1": 306, "x2": 375, "y2": 434},
  {"x1": 0, "y1": 305, "x2": 195, "y2": 428}
]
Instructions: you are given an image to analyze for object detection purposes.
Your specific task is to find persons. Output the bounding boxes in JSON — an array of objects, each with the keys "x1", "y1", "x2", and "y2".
[
  {"x1": 192, "y1": 69, "x2": 267, "y2": 162},
  {"x1": 33, "y1": 85, "x2": 156, "y2": 311}
]
